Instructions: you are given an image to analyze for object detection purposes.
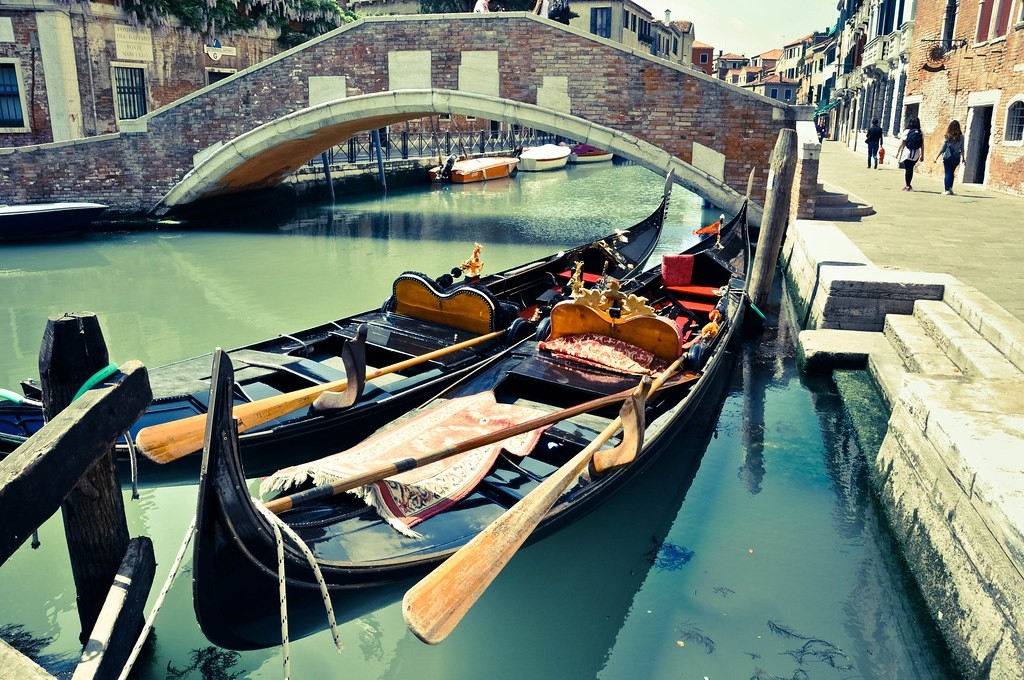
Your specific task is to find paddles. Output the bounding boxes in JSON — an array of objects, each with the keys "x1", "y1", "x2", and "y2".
[
  {"x1": 263, "y1": 370, "x2": 702, "y2": 510},
  {"x1": 402, "y1": 352, "x2": 688, "y2": 646},
  {"x1": 135, "y1": 329, "x2": 509, "y2": 465}
]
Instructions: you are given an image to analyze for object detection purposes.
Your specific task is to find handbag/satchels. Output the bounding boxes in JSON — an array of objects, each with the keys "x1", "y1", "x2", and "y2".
[
  {"x1": 865, "y1": 127, "x2": 872, "y2": 144},
  {"x1": 943, "y1": 146, "x2": 953, "y2": 161},
  {"x1": 879, "y1": 148, "x2": 883, "y2": 164}
]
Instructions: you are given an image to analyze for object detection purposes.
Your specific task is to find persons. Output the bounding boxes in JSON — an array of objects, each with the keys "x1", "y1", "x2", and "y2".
[
  {"x1": 817, "y1": 120, "x2": 826, "y2": 144},
  {"x1": 474, "y1": 0, "x2": 491, "y2": 13},
  {"x1": 895, "y1": 115, "x2": 924, "y2": 191},
  {"x1": 865, "y1": 118, "x2": 883, "y2": 169},
  {"x1": 933, "y1": 120, "x2": 966, "y2": 194}
]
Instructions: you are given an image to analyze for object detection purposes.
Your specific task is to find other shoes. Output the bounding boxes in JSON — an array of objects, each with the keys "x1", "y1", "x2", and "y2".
[
  {"x1": 941, "y1": 190, "x2": 950, "y2": 194},
  {"x1": 908, "y1": 185, "x2": 912, "y2": 190},
  {"x1": 902, "y1": 187, "x2": 910, "y2": 192},
  {"x1": 949, "y1": 187, "x2": 953, "y2": 195}
]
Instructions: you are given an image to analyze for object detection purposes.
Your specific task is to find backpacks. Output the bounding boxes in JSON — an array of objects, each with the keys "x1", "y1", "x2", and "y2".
[{"x1": 904, "y1": 128, "x2": 923, "y2": 149}]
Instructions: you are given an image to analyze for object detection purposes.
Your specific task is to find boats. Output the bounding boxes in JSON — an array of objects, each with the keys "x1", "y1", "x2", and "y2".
[
  {"x1": 188, "y1": 169, "x2": 760, "y2": 646},
  {"x1": 517, "y1": 144, "x2": 571, "y2": 170},
  {"x1": 569, "y1": 150, "x2": 613, "y2": 162},
  {"x1": 0, "y1": 173, "x2": 673, "y2": 464},
  {"x1": 428, "y1": 156, "x2": 520, "y2": 183}
]
